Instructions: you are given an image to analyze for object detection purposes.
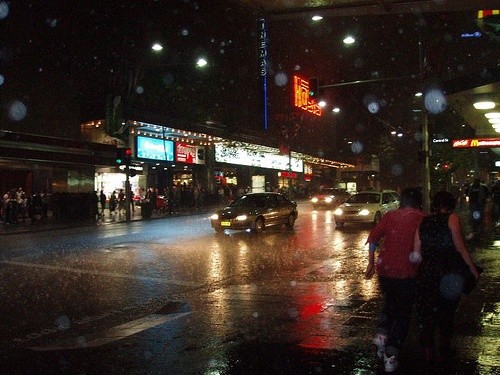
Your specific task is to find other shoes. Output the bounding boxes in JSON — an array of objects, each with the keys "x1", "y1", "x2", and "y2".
[
  {"x1": 438, "y1": 345, "x2": 457, "y2": 359},
  {"x1": 422, "y1": 347, "x2": 433, "y2": 365}
]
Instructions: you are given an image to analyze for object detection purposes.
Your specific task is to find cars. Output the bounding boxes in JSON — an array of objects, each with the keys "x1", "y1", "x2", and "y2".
[
  {"x1": 312, "y1": 187, "x2": 352, "y2": 210},
  {"x1": 334, "y1": 189, "x2": 403, "y2": 228},
  {"x1": 210, "y1": 193, "x2": 299, "y2": 234}
]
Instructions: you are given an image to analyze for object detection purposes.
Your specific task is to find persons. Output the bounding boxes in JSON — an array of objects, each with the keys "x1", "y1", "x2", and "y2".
[
  {"x1": 97, "y1": 189, "x2": 106, "y2": 218},
  {"x1": 163, "y1": 180, "x2": 212, "y2": 216},
  {"x1": 0, "y1": 179, "x2": 67, "y2": 228},
  {"x1": 273, "y1": 181, "x2": 327, "y2": 200},
  {"x1": 363, "y1": 189, "x2": 428, "y2": 374},
  {"x1": 109, "y1": 185, "x2": 118, "y2": 222},
  {"x1": 128, "y1": 186, "x2": 136, "y2": 213},
  {"x1": 452, "y1": 178, "x2": 500, "y2": 243},
  {"x1": 117, "y1": 190, "x2": 124, "y2": 212},
  {"x1": 413, "y1": 191, "x2": 481, "y2": 375}
]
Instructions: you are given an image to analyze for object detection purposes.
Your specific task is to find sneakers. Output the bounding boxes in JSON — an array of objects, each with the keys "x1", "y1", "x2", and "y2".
[
  {"x1": 371, "y1": 332, "x2": 387, "y2": 360},
  {"x1": 383, "y1": 352, "x2": 401, "y2": 372}
]
